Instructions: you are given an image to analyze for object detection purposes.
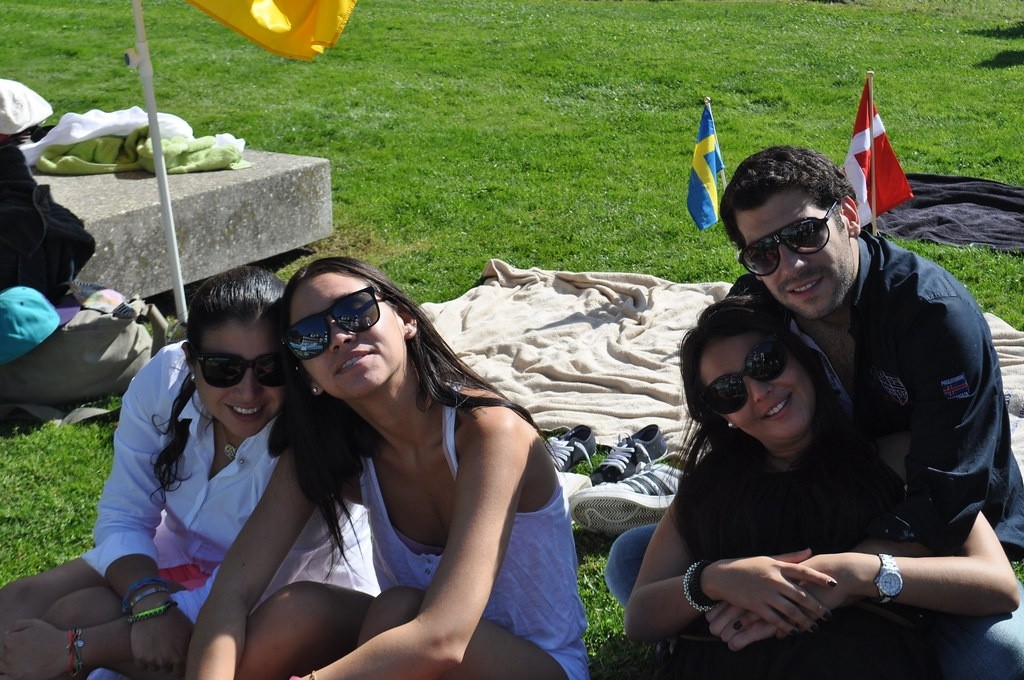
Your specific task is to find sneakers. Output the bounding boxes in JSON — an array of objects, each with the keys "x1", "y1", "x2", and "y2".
[
  {"x1": 543, "y1": 424, "x2": 596, "y2": 472},
  {"x1": 591, "y1": 424, "x2": 668, "y2": 486},
  {"x1": 568, "y1": 452, "x2": 698, "y2": 538}
]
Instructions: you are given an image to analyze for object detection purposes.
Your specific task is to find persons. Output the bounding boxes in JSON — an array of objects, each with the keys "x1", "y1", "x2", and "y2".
[
  {"x1": 623, "y1": 295, "x2": 1020, "y2": 679},
  {"x1": 605, "y1": 145, "x2": 1024, "y2": 680},
  {"x1": 0, "y1": 267, "x2": 384, "y2": 680},
  {"x1": 183, "y1": 256, "x2": 589, "y2": 680}
]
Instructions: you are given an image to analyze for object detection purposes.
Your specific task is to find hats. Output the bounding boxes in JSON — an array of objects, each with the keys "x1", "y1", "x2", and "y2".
[{"x1": 0, "y1": 286, "x2": 80, "y2": 367}]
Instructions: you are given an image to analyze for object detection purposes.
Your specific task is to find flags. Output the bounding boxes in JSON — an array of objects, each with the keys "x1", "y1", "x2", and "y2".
[
  {"x1": 840, "y1": 77, "x2": 914, "y2": 229},
  {"x1": 185, "y1": 0, "x2": 358, "y2": 62},
  {"x1": 686, "y1": 104, "x2": 724, "y2": 232}
]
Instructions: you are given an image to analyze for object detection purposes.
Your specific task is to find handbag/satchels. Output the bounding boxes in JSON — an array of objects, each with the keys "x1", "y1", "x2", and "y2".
[
  {"x1": 655, "y1": 597, "x2": 944, "y2": 680},
  {"x1": 0, "y1": 295, "x2": 169, "y2": 425}
]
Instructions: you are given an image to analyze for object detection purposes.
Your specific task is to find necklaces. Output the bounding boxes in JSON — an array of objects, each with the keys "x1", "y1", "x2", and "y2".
[{"x1": 216, "y1": 419, "x2": 238, "y2": 459}]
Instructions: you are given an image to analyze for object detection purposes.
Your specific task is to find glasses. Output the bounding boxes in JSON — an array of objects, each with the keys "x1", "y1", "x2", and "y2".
[
  {"x1": 699, "y1": 332, "x2": 788, "y2": 415},
  {"x1": 280, "y1": 286, "x2": 396, "y2": 360},
  {"x1": 187, "y1": 339, "x2": 288, "y2": 388},
  {"x1": 736, "y1": 199, "x2": 839, "y2": 277}
]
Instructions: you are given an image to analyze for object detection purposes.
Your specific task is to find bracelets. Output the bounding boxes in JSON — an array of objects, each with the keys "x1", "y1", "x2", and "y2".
[
  {"x1": 682, "y1": 559, "x2": 721, "y2": 612},
  {"x1": 127, "y1": 600, "x2": 180, "y2": 623},
  {"x1": 129, "y1": 586, "x2": 168, "y2": 609},
  {"x1": 65, "y1": 628, "x2": 84, "y2": 677},
  {"x1": 311, "y1": 671, "x2": 316, "y2": 680},
  {"x1": 122, "y1": 577, "x2": 168, "y2": 611}
]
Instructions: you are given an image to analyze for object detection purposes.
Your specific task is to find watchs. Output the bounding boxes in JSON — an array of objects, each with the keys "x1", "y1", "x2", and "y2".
[{"x1": 874, "y1": 553, "x2": 903, "y2": 603}]
